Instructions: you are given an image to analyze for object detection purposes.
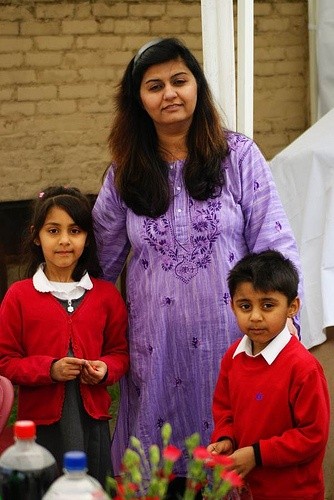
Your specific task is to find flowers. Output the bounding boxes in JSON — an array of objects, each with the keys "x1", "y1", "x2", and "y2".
[{"x1": 105, "y1": 422, "x2": 245, "y2": 500}]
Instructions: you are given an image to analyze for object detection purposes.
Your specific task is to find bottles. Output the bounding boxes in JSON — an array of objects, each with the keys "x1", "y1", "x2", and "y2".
[
  {"x1": 0, "y1": 421, "x2": 61, "y2": 500},
  {"x1": 42, "y1": 451, "x2": 111, "y2": 500}
]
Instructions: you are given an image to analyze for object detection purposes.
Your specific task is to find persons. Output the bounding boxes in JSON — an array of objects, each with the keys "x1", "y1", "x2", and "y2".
[
  {"x1": 90, "y1": 36, "x2": 302, "y2": 486},
  {"x1": 205, "y1": 249, "x2": 330, "y2": 500},
  {"x1": 0, "y1": 187, "x2": 131, "y2": 500}
]
